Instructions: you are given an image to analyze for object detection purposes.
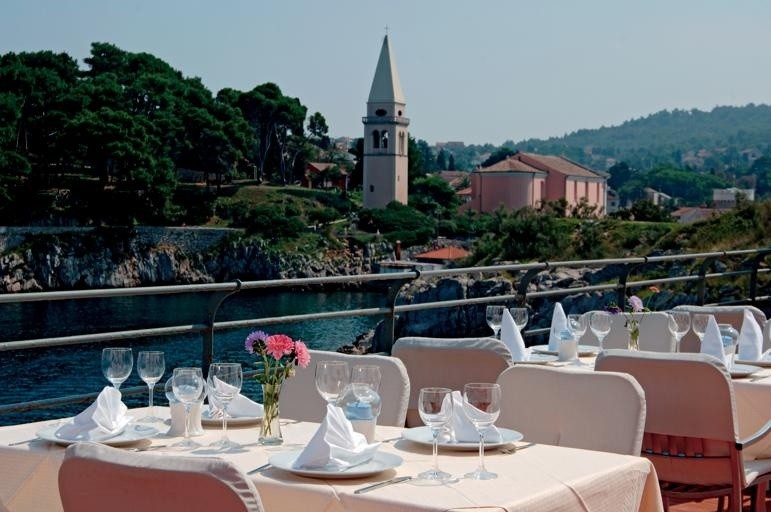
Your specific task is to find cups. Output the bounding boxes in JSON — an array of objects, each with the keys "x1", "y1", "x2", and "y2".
[
  {"x1": 313, "y1": 359, "x2": 350, "y2": 406},
  {"x1": 351, "y1": 364, "x2": 382, "y2": 407}
]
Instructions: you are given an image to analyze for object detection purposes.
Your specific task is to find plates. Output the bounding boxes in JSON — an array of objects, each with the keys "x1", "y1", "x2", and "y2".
[
  {"x1": 734, "y1": 352, "x2": 771, "y2": 365},
  {"x1": 407, "y1": 425, "x2": 522, "y2": 450},
  {"x1": 719, "y1": 362, "x2": 764, "y2": 377},
  {"x1": 198, "y1": 416, "x2": 266, "y2": 425},
  {"x1": 514, "y1": 353, "x2": 559, "y2": 365},
  {"x1": 532, "y1": 342, "x2": 596, "y2": 355},
  {"x1": 37, "y1": 420, "x2": 162, "y2": 447},
  {"x1": 269, "y1": 448, "x2": 402, "y2": 478}
]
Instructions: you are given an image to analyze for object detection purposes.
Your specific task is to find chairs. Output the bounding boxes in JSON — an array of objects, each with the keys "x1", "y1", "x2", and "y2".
[
  {"x1": 593, "y1": 351, "x2": 771, "y2": 512},
  {"x1": 479, "y1": 364, "x2": 646, "y2": 461},
  {"x1": 389, "y1": 337, "x2": 513, "y2": 429},
  {"x1": 52, "y1": 442, "x2": 269, "y2": 512},
  {"x1": 271, "y1": 347, "x2": 409, "y2": 429}
]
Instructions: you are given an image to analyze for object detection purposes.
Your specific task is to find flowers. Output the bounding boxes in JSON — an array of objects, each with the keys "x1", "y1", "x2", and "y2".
[
  {"x1": 605, "y1": 283, "x2": 657, "y2": 319},
  {"x1": 243, "y1": 328, "x2": 310, "y2": 434}
]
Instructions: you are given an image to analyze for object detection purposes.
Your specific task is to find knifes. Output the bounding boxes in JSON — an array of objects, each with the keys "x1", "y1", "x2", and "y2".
[
  {"x1": 501, "y1": 439, "x2": 537, "y2": 456},
  {"x1": 353, "y1": 475, "x2": 412, "y2": 497},
  {"x1": 138, "y1": 443, "x2": 168, "y2": 452}
]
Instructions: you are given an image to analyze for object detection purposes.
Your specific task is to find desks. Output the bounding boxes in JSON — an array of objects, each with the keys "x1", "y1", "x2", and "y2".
[
  {"x1": 506, "y1": 338, "x2": 771, "y2": 473},
  {"x1": 672, "y1": 302, "x2": 766, "y2": 354}
]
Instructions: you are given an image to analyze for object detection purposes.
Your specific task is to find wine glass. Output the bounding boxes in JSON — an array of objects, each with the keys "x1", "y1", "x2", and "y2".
[
  {"x1": 667, "y1": 310, "x2": 692, "y2": 351},
  {"x1": 462, "y1": 384, "x2": 503, "y2": 480},
  {"x1": 568, "y1": 312, "x2": 612, "y2": 354},
  {"x1": 415, "y1": 387, "x2": 460, "y2": 487},
  {"x1": 102, "y1": 347, "x2": 136, "y2": 388},
  {"x1": 136, "y1": 351, "x2": 167, "y2": 422},
  {"x1": 486, "y1": 305, "x2": 529, "y2": 339},
  {"x1": 172, "y1": 365, "x2": 203, "y2": 449},
  {"x1": 692, "y1": 313, "x2": 716, "y2": 351},
  {"x1": 207, "y1": 363, "x2": 244, "y2": 449}
]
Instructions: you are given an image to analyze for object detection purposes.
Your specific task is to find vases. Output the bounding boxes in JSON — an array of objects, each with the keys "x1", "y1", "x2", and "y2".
[
  {"x1": 256, "y1": 383, "x2": 285, "y2": 448},
  {"x1": 622, "y1": 316, "x2": 643, "y2": 351}
]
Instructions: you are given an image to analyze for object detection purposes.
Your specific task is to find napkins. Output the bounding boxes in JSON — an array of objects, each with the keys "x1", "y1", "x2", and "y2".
[
  {"x1": 548, "y1": 300, "x2": 575, "y2": 348},
  {"x1": 738, "y1": 308, "x2": 763, "y2": 362},
  {"x1": 499, "y1": 312, "x2": 530, "y2": 361},
  {"x1": 702, "y1": 314, "x2": 727, "y2": 373}
]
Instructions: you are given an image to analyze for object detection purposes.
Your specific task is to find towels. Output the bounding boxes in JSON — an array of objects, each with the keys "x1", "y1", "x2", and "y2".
[
  {"x1": 208, "y1": 374, "x2": 262, "y2": 419},
  {"x1": 289, "y1": 403, "x2": 382, "y2": 475},
  {"x1": 432, "y1": 389, "x2": 500, "y2": 444},
  {"x1": 52, "y1": 386, "x2": 134, "y2": 442}
]
